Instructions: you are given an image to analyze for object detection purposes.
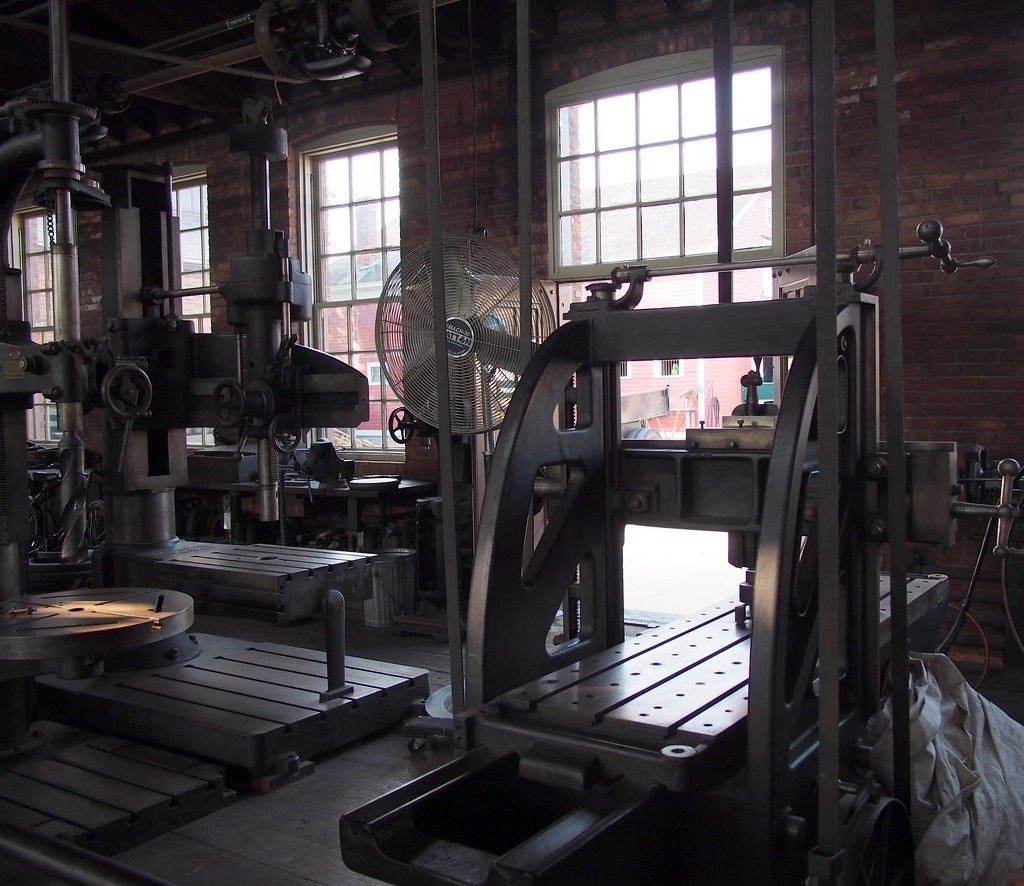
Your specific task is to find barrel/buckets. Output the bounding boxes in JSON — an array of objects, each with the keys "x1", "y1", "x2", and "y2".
[{"x1": 364, "y1": 547, "x2": 419, "y2": 628}]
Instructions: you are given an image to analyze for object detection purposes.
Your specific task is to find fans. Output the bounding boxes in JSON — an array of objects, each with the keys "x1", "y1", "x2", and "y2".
[{"x1": 374, "y1": 233, "x2": 556, "y2": 717}]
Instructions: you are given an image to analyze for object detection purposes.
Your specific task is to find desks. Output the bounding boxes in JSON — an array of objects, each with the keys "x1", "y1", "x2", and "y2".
[{"x1": 179, "y1": 476, "x2": 434, "y2": 551}]
